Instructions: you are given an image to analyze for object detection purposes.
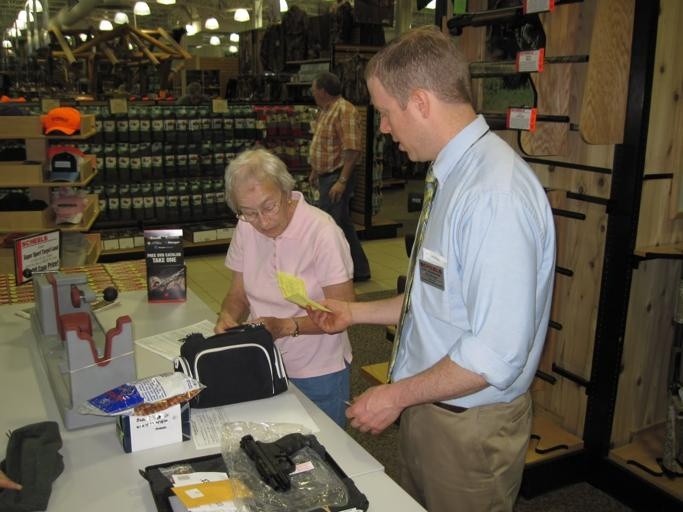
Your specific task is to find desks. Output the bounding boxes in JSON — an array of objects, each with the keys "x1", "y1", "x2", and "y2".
[{"x1": 1, "y1": 287, "x2": 426, "y2": 512}]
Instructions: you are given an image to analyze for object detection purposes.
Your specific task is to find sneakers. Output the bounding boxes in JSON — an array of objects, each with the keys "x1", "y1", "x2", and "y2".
[{"x1": 353, "y1": 272, "x2": 370, "y2": 283}]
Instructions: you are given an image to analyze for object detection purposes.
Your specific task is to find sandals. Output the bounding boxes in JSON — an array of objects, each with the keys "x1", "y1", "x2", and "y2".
[
  {"x1": 128, "y1": 95, "x2": 155, "y2": 104},
  {"x1": 158, "y1": 97, "x2": 175, "y2": 106}
]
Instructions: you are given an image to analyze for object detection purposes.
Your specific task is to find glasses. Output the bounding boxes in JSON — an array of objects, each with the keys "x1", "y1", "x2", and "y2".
[{"x1": 237, "y1": 192, "x2": 281, "y2": 222}]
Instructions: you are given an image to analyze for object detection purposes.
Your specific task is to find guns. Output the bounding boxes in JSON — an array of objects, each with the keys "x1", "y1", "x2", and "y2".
[{"x1": 240, "y1": 433, "x2": 303, "y2": 491}]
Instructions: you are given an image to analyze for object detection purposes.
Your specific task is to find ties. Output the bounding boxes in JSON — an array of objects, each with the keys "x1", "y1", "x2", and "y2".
[{"x1": 385, "y1": 165, "x2": 438, "y2": 383}]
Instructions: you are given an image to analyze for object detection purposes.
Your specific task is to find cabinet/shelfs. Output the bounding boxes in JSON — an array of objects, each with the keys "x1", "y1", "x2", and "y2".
[{"x1": 359, "y1": 0, "x2": 683, "y2": 511}]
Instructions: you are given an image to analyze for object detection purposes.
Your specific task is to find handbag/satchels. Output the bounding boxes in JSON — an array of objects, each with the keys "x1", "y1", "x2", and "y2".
[{"x1": 173, "y1": 322, "x2": 289, "y2": 408}]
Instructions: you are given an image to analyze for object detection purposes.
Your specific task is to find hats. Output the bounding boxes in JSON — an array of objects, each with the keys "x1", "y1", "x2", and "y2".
[
  {"x1": 53, "y1": 195, "x2": 85, "y2": 225},
  {"x1": 61, "y1": 231, "x2": 90, "y2": 268},
  {"x1": 44, "y1": 107, "x2": 80, "y2": 136},
  {"x1": 49, "y1": 151, "x2": 80, "y2": 182}
]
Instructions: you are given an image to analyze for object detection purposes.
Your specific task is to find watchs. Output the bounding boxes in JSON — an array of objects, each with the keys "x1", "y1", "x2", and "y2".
[{"x1": 290, "y1": 317, "x2": 299, "y2": 337}]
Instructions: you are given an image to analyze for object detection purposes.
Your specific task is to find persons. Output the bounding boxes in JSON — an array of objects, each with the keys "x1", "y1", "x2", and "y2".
[
  {"x1": 174, "y1": 81, "x2": 211, "y2": 105},
  {"x1": 0, "y1": 469, "x2": 24, "y2": 491},
  {"x1": 305, "y1": 25, "x2": 556, "y2": 512},
  {"x1": 209, "y1": 76, "x2": 220, "y2": 96},
  {"x1": 308, "y1": 71, "x2": 372, "y2": 284},
  {"x1": 214, "y1": 143, "x2": 356, "y2": 431}
]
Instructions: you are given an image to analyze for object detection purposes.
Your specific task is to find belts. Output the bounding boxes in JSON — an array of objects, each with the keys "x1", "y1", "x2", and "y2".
[{"x1": 433, "y1": 399, "x2": 467, "y2": 413}]
